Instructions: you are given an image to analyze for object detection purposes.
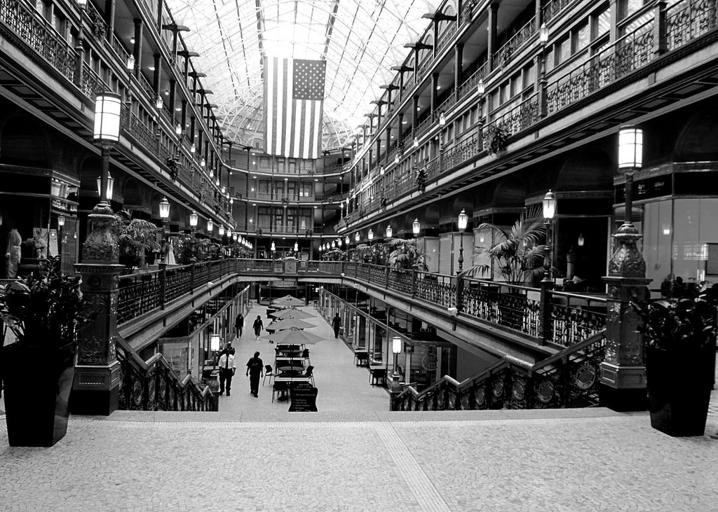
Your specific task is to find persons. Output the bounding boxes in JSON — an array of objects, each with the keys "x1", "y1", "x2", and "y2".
[
  {"x1": 217, "y1": 348, "x2": 236, "y2": 398},
  {"x1": 252, "y1": 314, "x2": 264, "y2": 341},
  {"x1": 220, "y1": 341, "x2": 235, "y2": 355},
  {"x1": 246, "y1": 351, "x2": 264, "y2": 398},
  {"x1": 331, "y1": 312, "x2": 342, "y2": 339},
  {"x1": 234, "y1": 313, "x2": 244, "y2": 341}
]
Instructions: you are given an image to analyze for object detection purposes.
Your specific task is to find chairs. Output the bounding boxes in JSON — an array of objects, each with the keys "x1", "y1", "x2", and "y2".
[
  {"x1": 263, "y1": 344, "x2": 314, "y2": 403},
  {"x1": 354, "y1": 346, "x2": 387, "y2": 386}
]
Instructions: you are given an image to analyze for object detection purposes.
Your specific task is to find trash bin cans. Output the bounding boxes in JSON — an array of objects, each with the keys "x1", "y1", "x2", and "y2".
[
  {"x1": 202, "y1": 360, "x2": 219, "y2": 392},
  {"x1": 274, "y1": 261, "x2": 283, "y2": 274}
]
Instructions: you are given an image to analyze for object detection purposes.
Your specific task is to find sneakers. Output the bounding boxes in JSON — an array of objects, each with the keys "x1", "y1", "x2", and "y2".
[{"x1": 219, "y1": 389, "x2": 258, "y2": 398}]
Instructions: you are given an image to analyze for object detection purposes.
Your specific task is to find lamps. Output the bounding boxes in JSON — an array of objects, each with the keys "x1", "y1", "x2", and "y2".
[
  {"x1": 331, "y1": 240, "x2": 335, "y2": 248},
  {"x1": 222, "y1": 187, "x2": 225, "y2": 193},
  {"x1": 232, "y1": 232, "x2": 253, "y2": 249},
  {"x1": 127, "y1": 54, "x2": 135, "y2": 71},
  {"x1": 216, "y1": 180, "x2": 220, "y2": 186},
  {"x1": 340, "y1": 166, "x2": 385, "y2": 209},
  {"x1": 542, "y1": 191, "x2": 558, "y2": 225},
  {"x1": 230, "y1": 197, "x2": 233, "y2": 204},
  {"x1": 175, "y1": 123, "x2": 182, "y2": 135},
  {"x1": 93, "y1": 92, "x2": 124, "y2": 146},
  {"x1": 392, "y1": 336, "x2": 402, "y2": 375},
  {"x1": 337, "y1": 238, "x2": 342, "y2": 246},
  {"x1": 355, "y1": 232, "x2": 361, "y2": 242},
  {"x1": 478, "y1": 78, "x2": 485, "y2": 97},
  {"x1": 438, "y1": 113, "x2": 447, "y2": 127},
  {"x1": 201, "y1": 158, "x2": 205, "y2": 166},
  {"x1": 210, "y1": 333, "x2": 220, "y2": 375},
  {"x1": 157, "y1": 197, "x2": 171, "y2": 223},
  {"x1": 227, "y1": 229, "x2": 232, "y2": 238},
  {"x1": 615, "y1": 124, "x2": 644, "y2": 175},
  {"x1": 190, "y1": 143, "x2": 196, "y2": 153},
  {"x1": 457, "y1": 208, "x2": 468, "y2": 233},
  {"x1": 210, "y1": 170, "x2": 214, "y2": 177},
  {"x1": 318, "y1": 244, "x2": 326, "y2": 251},
  {"x1": 345, "y1": 236, "x2": 350, "y2": 244},
  {"x1": 226, "y1": 193, "x2": 230, "y2": 199},
  {"x1": 394, "y1": 153, "x2": 400, "y2": 164},
  {"x1": 207, "y1": 219, "x2": 214, "y2": 234},
  {"x1": 540, "y1": 22, "x2": 549, "y2": 46},
  {"x1": 368, "y1": 229, "x2": 374, "y2": 240},
  {"x1": 96, "y1": 171, "x2": 114, "y2": 203},
  {"x1": 188, "y1": 212, "x2": 200, "y2": 230},
  {"x1": 219, "y1": 225, "x2": 225, "y2": 236},
  {"x1": 156, "y1": 94, "x2": 163, "y2": 109},
  {"x1": 386, "y1": 224, "x2": 393, "y2": 238},
  {"x1": 326, "y1": 242, "x2": 331, "y2": 249},
  {"x1": 271, "y1": 243, "x2": 276, "y2": 251},
  {"x1": 294, "y1": 243, "x2": 299, "y2": 251},
  {"x1": 412, "y1": 218, "x2": 421, "y2": 235},
  {"x1": 413, "y1": 135, "x2": 419, "y2": 148},
  {"x1": 77, "y1": 0, "x2": 87, "y2": 12}
]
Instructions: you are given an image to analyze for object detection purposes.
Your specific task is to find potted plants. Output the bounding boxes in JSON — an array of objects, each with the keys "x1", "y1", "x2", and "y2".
[
  {"x1": 0, "y1": 266, "x2": 92, "y2": 448},
  {"x1": 455, "y1": 204, "x2": 551, "y2": 331},
  {"x1": 628, "y1": 272, "x2": 718, "y2": 438}
]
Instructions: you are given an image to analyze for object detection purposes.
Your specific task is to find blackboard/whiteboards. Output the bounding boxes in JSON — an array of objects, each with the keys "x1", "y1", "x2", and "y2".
[{"x1": 289, "y1": 381, "x2": 318, "y2": 412}]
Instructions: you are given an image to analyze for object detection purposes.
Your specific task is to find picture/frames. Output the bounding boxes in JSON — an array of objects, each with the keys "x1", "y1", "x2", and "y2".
[{"x1": 705, "y1": 242, "x2": 718, "y2": 275}]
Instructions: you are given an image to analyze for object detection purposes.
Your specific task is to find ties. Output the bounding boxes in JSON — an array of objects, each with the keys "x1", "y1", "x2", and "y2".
[{"x1": 225, "y1": 356, "x2": 229, "y2": 369}]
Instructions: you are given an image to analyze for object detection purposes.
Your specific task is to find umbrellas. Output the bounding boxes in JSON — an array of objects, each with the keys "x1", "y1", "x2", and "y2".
[
  {"x1": 271, "y1": 294, "x2": 305, "y2": 309},
  {"x1": 261, "y1": 328, "x2": 327, "y2": 369},
  {"x1": 268, "y1": 304, "x2": 320, "y2": 321},
  {"x1": 265, "y1": 314, "x2": 318, "y2": 357}
]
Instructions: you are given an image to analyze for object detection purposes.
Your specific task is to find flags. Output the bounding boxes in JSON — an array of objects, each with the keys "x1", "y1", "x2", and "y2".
[{"x1": 261, "y1": 54, "x2": 328, "y2": 163}]
